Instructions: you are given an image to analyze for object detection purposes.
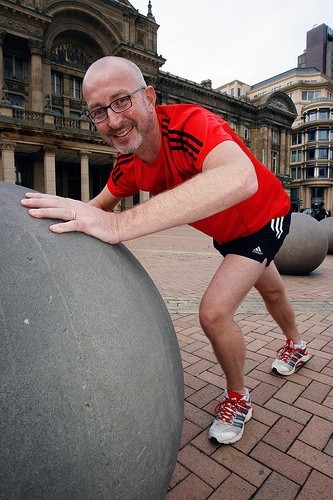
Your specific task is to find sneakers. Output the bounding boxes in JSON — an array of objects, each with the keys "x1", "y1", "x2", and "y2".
[
  {"x1": 208, "y1": 386, "x2": 254, "y2": 445},
  {"x1": 271, "y1": 339, "x2": 310, "y2": 376}
]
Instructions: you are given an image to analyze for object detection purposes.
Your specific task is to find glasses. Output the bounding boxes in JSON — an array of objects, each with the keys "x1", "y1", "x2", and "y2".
[{"x1": 86, "y1": 84, "x2": 146, "y2": 124}]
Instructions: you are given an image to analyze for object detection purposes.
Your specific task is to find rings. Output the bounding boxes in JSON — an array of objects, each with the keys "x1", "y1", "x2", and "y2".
[{"x1": 71, "y1": 209, "x2": 76, "y2": 220}]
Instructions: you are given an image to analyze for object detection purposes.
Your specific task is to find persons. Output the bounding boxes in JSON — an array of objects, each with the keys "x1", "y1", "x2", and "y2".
[{"x1": 19, "y1": 56, "x2": 310, "y2": 445}]
